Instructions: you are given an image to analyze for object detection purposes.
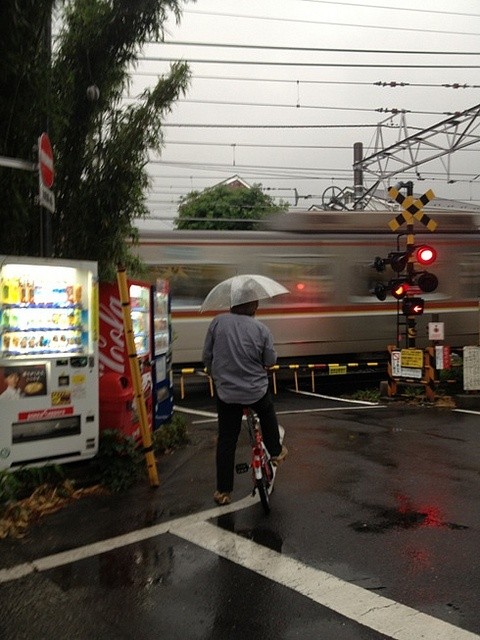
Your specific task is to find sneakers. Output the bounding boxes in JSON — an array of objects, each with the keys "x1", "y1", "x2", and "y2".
[
  {"x1": 214, "y1": 490, "x2": 230, "y2": 504},
  {"x1": 270, "y1": 445, "x2": 287, "y2": 466}
]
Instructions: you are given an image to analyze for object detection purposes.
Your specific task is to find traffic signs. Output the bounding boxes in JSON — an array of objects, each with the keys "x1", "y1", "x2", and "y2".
[{"x1": 39, "y1": 186, "x2": 56, "y2": 212}]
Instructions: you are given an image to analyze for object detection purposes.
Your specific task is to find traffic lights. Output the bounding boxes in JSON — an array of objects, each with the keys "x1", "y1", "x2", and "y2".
[
  {"x1": 416, "y1": 246, "x2": 437, "y2": 265},
  {"x1": 418, "y1": 273, "x2": 437, "y2": 291},
  {"x1": 402, "y1": 295, "x2": 424, "y2": 316},
  {"x1": 391, "y1": 253, "x2": 409, "y2": 273},
  {"x1": 392, "y1": 281, "x2": 409, "y2": 300}
]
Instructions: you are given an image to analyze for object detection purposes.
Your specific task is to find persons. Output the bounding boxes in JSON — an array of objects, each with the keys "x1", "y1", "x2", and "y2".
[
  {"x1": 203, "y1": 289, "x2": 288, "y2": 507},
  {"x1": 0, "y1": 369, "x2": 27, "y2": 400}
]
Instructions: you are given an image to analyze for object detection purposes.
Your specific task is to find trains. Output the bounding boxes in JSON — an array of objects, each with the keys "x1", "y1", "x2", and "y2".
[{"x1": 113, "y1": 209, "x2": 480, "y2": 378}]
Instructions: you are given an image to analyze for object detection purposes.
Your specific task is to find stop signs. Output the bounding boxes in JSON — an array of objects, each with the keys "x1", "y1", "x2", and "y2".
[{"x1": 39, "y1": 134, "x2": 54, "y2": 185}]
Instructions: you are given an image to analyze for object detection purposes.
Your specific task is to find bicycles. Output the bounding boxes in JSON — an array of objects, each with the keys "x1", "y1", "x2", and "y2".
[{"x1": 235, "y1": 410, "x2": 284, "y2": 508}]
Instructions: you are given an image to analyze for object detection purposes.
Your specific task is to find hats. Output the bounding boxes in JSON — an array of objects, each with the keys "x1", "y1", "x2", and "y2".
[{"x1": 230, "y1": 290, "x2": 258, "y2": 314}]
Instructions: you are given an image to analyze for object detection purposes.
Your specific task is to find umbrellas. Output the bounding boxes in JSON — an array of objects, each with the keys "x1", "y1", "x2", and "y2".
[{"x1": 198, "y1": 273, "x2": 290, "y2": 315}]
